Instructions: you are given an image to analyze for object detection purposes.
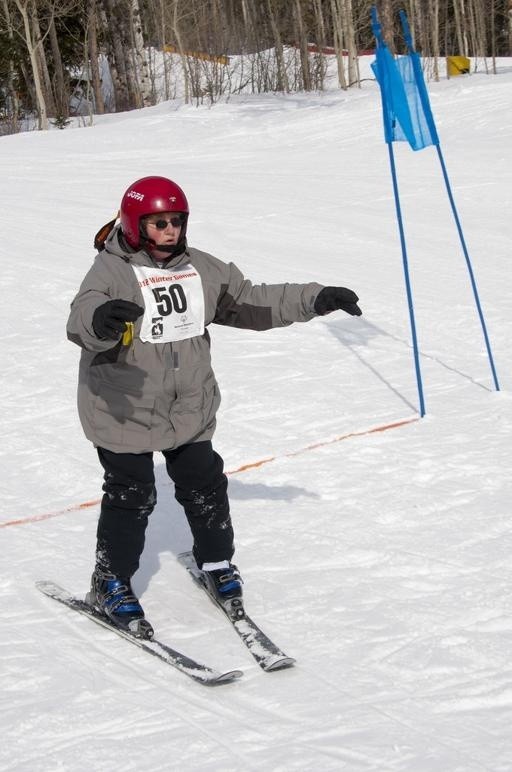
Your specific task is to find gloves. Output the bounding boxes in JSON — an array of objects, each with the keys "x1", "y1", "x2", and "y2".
[
  {"x1": 315, "y1": 284, "x2": 362, "y2": 318},
  {"x1": 92, "y1": 299, "x2": 144, "y2": 340}
]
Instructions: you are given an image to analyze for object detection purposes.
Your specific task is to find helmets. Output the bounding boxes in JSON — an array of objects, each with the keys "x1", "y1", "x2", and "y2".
[{"x1": 120, "y1": 176, "x2": 189, "y2": 252}]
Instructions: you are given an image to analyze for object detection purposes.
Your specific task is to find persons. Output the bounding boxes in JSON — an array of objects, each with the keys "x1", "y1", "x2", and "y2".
[{"x1": 59, "y1": 174, "x2": 366, "y2": 622}]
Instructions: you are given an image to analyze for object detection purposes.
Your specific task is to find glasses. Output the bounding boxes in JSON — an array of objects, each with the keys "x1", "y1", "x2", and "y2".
[{"x1": 148, "y1": 218, "x2": 183, "y2": 228}]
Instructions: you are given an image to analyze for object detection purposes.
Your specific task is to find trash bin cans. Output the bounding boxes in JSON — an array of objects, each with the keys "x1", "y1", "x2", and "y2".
[{"x1": 447, "y1": 56, "x2": 470, "y2": 80}]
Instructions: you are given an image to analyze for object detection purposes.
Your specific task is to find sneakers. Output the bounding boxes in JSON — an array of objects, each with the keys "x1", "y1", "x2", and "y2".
[
  {"x1": 206, "y1": 564, "x2": 242, "y2": 599},
  {"x1": 91, "y1": 564, "x2": 145, "y2": 617}
]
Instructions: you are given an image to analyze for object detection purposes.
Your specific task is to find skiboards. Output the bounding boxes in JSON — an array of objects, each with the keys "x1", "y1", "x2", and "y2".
[{"x1": 35, "y1": 551, "x2": 296, "y2": 687}]
